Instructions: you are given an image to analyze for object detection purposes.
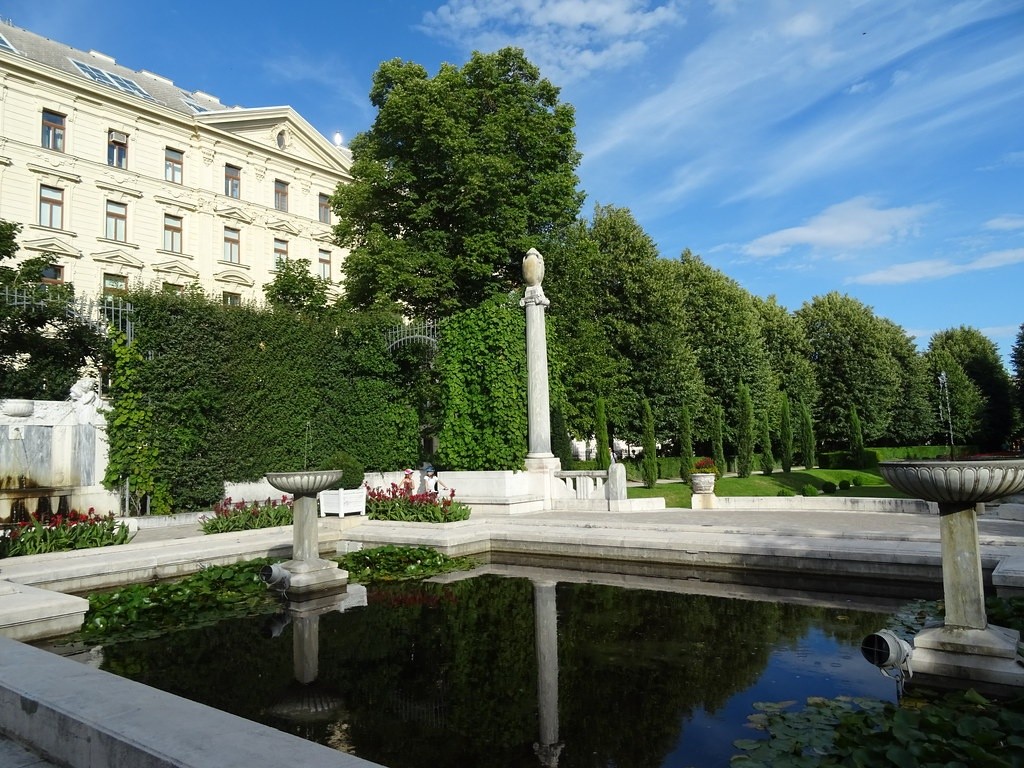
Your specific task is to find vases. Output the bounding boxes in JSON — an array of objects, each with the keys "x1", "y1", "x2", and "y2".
[{"x1": 690, "y1": 473, "x2": 716, "y2": 493}]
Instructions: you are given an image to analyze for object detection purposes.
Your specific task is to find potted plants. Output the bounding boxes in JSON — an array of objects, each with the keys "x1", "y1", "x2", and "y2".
[{"x1": 317, "y1": 452, "x2": 368, "y2": 518}]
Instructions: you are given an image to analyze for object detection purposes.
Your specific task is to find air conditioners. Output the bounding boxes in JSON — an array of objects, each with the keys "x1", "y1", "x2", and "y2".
[{"x1": 110, "y1": 131, "x2": 127, "y2": 144}]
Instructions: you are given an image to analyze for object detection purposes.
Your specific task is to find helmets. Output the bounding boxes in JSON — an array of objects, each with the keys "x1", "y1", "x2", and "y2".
[
  {"x1": 426, "y1": 466, "x2": 435, "y2": 472},
  {"x1": 405, "y1": 468, "x2": 414, "y2": 475}
]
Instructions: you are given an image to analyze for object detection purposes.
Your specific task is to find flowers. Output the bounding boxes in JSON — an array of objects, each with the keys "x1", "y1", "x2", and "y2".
[{"x1": 690, "y1": 456, "x2": 718, "y2": 474}]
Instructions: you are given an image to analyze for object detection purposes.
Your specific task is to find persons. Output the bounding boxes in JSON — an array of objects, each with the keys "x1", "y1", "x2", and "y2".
[
  {"x1": 398, "y1": 469, "x2": 415, "y2": 498},
  {"x1": 423, "y1": 466, "x2": 449, "y2": 501}
]
